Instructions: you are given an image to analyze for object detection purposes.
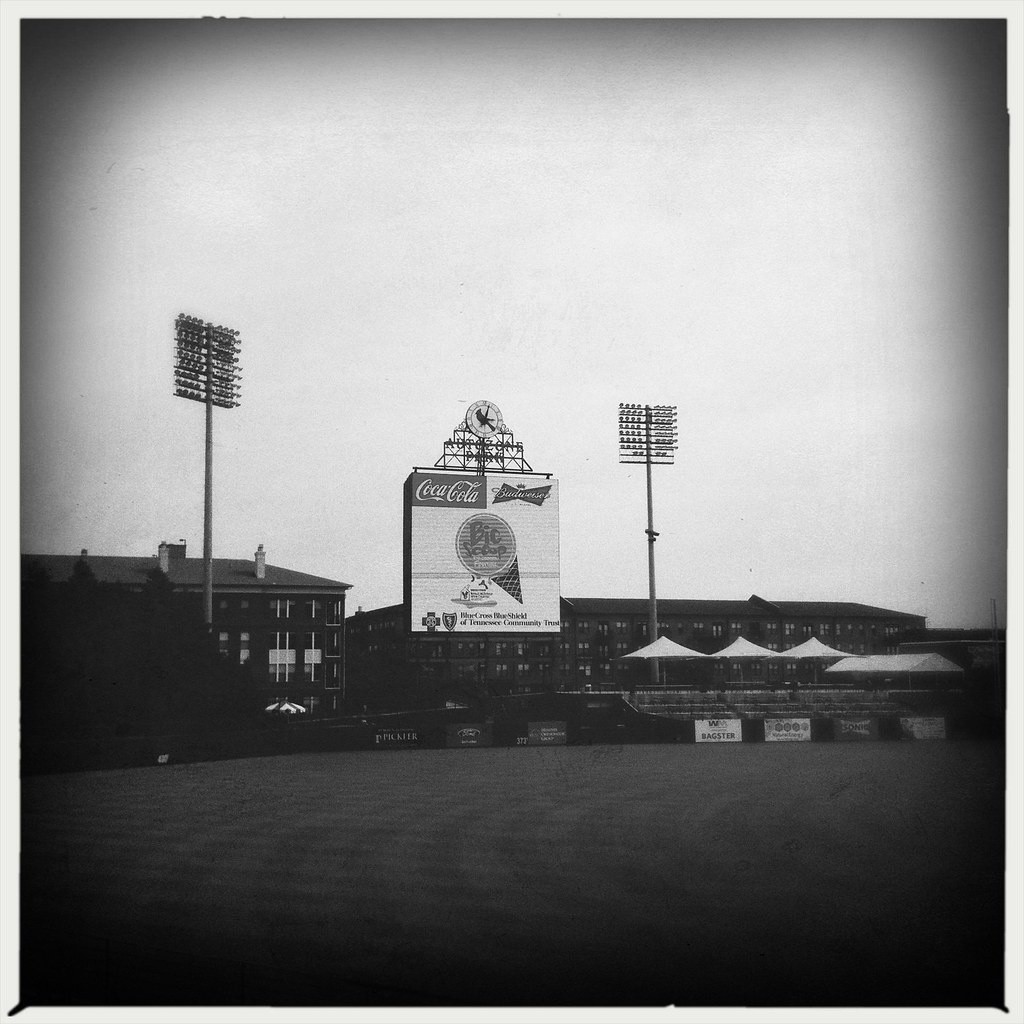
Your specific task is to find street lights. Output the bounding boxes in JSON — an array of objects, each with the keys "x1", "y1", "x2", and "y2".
[
  {"x1": 618, "y1": 402, "x2": 679, "y2": 685},
  {"x1": 171, "y1": 313, "x2": 242, "y2": 634}
]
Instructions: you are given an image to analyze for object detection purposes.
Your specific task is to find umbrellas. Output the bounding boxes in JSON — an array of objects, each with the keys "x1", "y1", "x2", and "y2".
[
  {"x1": 264, "y1": 701, "x2": 306, "y2": 714},
  {"x1": 615, "y1": 636, "x2": 715, "y2": 686},
  {"x1": 772, "y1": 637, "x2": 857, "y2": 684},
  {"x1": 686, "y1": 636, "x2": 801, "y2": 690}
]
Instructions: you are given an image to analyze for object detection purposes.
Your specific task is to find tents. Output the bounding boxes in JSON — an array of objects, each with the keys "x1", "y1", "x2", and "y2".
[{"x1": 825, "y1": 652, "x2": 965, "y2": 690}]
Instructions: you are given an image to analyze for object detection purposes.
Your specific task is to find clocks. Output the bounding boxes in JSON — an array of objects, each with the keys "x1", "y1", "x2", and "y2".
[{"x1": 466, "y1": 400, "x2": 503, "y2": 438}]
